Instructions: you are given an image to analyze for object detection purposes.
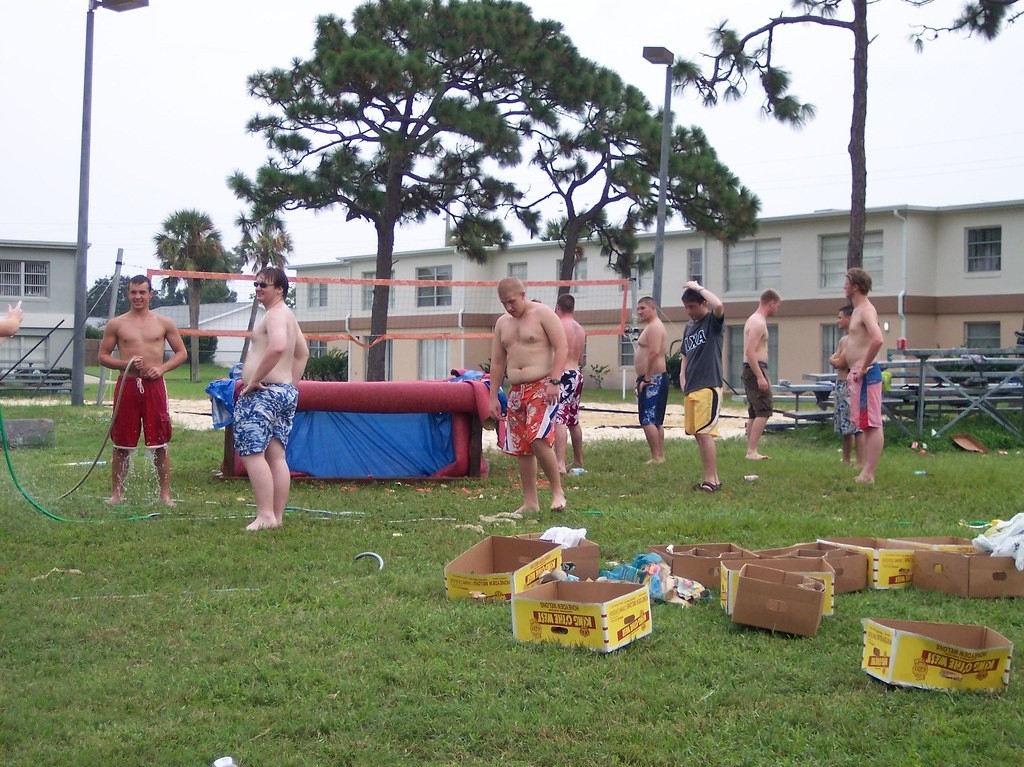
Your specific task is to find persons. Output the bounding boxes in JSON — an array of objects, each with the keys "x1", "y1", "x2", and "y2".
[
  {"x1": 741, "y1": 290, "x2": 781, "y2": 460},
  {"x1": 679, "y1": 280, "x2": 725, "y2": 492},
  {"x1": 0, "y1": 300, "x2": 24, "y2": 345},
  {"x1": 829, "y1": 267, "x2": 884, "y2": 484},
  {"x1": 232, "y1": 267, "x2": 309, "y2": 531},
  {"x1": 635, "y1": 296, "x2": 670, "y2": 464},
  {"x1": 489, "y1": 277, "x2": 584, "y2": 519},
  {"x1": 98, "y1": 275, "x2": 188, "y2": 508}
]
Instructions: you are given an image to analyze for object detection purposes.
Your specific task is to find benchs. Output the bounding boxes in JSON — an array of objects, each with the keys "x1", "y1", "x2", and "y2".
[
  {"x1": 730, "y1": 346, "x2": 1024, "y2": 447},
  {"x1": 0, "y1": 359, "x2": 73, "y2": 396}
]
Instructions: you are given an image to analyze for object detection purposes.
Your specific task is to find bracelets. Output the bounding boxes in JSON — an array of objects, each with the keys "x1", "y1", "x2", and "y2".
[{"x1": 643, "y1": 380, "x2": 651, "y2": 383}]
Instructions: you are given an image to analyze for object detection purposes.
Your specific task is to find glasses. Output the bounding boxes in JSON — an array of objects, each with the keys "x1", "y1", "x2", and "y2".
[{"x1": 254, "y1": 281, "x2": 275, "y2": 288}]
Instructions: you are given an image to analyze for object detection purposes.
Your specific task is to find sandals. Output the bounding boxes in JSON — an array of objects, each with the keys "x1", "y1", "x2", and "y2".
[{"x1": 694, "y1": 481, "x2": 722, "y2": 494}]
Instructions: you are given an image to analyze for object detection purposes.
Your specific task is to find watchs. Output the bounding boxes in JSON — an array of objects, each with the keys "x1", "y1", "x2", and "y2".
[
  {"x1": 697, "y1": 286, "x2": 705, "y2": 293},
  {"x1": 550, "y1": 379, "x2": 560, "y2": 385}
]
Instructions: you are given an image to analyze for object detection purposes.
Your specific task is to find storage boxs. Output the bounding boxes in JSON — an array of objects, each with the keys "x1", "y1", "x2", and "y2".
[
  {"x1": 511, "y1": 579, "x2": 652, "y2": 653},
  {"x1": 754, "y1": 542, "x2": 866, "y2": 595},
  {"x1": 646, "y1": 543, "x2": 760, "y2": 588},
  {"x1": 912, "y1": 550, "x2": 1024, "y2": 599},
  {"x1": 506, "y1": 531, "x2": 599, "y2": 581},
  {"x1": 444, "y1": 534, "x2": 562, "y2": 604},
  {"x1": 817, "y1": 537, "x2": 934, "y2": 590},
  {"x1": 730, "y1": 563, "x2": 826, "y2": 637},
  {"x1": 887, "y1": 535, "x2": 981, "y2": 551},
  {"x1": 720, "y1": 557, "x2": 836, "y2": 617},
  {"x1": 860, "y1": 618, "x2": 1015, "y2": 694}
]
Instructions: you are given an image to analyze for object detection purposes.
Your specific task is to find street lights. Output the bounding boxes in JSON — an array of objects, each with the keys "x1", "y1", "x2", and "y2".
[
  {"x1": 70, "y1": 1, "x2": 150, "y2": 408},
  {"x1": 641, "y1": 44, "x2": 673, "y2": 319}
]
